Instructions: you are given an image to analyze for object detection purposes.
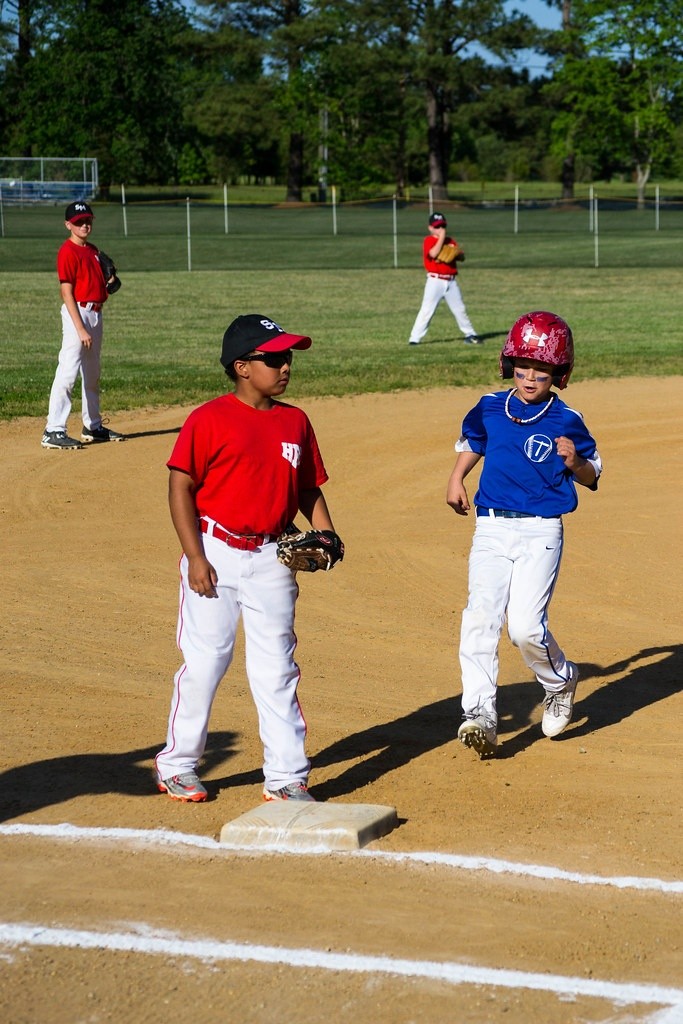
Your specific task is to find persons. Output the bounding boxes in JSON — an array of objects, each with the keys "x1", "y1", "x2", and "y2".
[
  {"x1": 40, "y1": 201, "x2": 127, "y2": 450},
  {"x1": 560, "y1": 155, "x2": 576, "y2": 200},
  {"x1": 408, "y1": 213, "x2": 480, "y2": 346},
  {"x1": 447, "y1": 310, "x2": 604, "y2": 760},
  {"x1": 152, "y1": 316, "x2": 345, "y2": 803}
]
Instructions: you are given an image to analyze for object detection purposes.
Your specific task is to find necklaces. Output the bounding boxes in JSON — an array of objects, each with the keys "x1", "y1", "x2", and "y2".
[{"x1": 505, "y1": 388, "x2": 554, "y2": 424}]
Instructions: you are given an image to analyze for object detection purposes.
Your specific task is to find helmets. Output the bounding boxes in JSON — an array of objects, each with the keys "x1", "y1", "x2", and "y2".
[{"x1": 499, "y1": 311, "x2": 575, "y2": 390}]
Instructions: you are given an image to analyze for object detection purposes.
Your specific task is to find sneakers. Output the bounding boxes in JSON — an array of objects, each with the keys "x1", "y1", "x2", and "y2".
[
  {"x1": 262, "y1": 779, "x2": 315, "y2": 804},
  {"x1": 540, "y1": 659, "x2": 579, "y2": 737},
  {"x1": 41, "y1": 428, "x2": 83, "y2": 449},
  {"x1": 80, "y1": 417, "x2": 125, "y2": 442},
  {"x1": 158, "y1": 769, "x2": 207, "y2": 802},
  {"x1": 458, "y1": 714, "x2": 499, "y2": 759}
]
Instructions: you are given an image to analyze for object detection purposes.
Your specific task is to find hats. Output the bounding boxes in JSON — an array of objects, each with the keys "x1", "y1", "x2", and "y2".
[
  {"x1": 65, "y1": 203, "x2": 96, "y2": 224},
  {"x1": 429, "y1": 213, "x2": 447, "y2": 226},
  {"x1": 220, "y1": 313, "x2": 312, "y2": 370}
]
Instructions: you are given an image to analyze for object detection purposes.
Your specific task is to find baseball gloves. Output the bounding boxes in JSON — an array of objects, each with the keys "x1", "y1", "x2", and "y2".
[
  {"x1": 97, "y1": 250, "x2": 118, "y2": 282},
  {"x1": 436, "y1": 245, "x2": 462, "y2": 263},
  {"x1": 274, "y1": 530, "x2": 346, "y2": 574}
]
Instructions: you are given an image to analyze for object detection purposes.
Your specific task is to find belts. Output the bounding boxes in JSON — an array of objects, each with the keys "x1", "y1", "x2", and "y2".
[
  {"x1": 477, "y1": 506, "x2": 535, "y2": 519},
  {"x1": 80, "y1": 300, "x2": 104, "y2": 313},
  {"x1": 431, "y1": 274, "x2": 451, "y2": 281},
  {"x1": 199, "y1": 518, "x2": 280, "y2": 552}
]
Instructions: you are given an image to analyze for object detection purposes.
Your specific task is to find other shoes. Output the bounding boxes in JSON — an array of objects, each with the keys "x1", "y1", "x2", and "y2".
[{"x1": 466, "y1": 337, "x2": 484, "y2": 346}]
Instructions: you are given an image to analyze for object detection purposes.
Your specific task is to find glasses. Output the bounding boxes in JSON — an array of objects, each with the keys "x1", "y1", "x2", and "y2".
[
  {"x1": 433, "y1": 224, "x2": 446, "y2": 228},
  {"x1": 236, "y1": 351, "x2": 293, "y2": 369},
  {"x1": 73, "y1": 218, "x2": 92, "y2": 227}
]
